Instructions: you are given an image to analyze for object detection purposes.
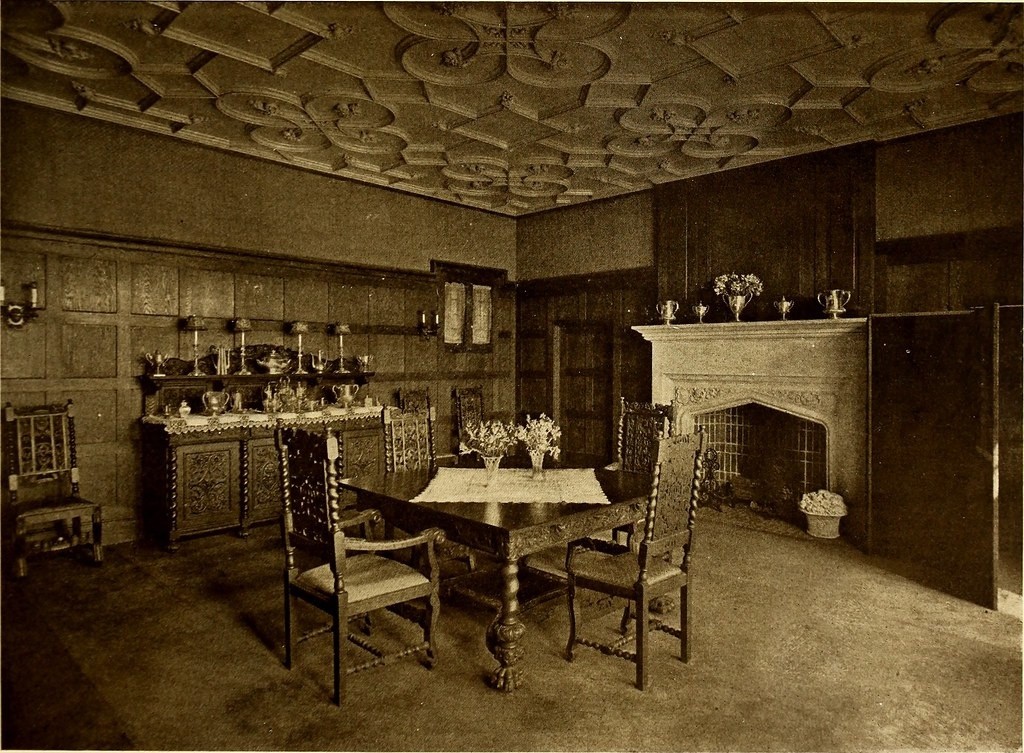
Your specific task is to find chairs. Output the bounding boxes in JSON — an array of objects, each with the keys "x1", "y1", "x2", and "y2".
[
  {"x1": 274, "y1": 416, "x2": 446, "y2": 707},
  {"x1": 5, "y1": 396, "x2": 104, "y2": 582},
  {"x1": 375, "y1": 405, "x2": 477, "y2": 574},
  {"x1": 611, "y1": 395, "x2": 676, "y2": 550},
  {"x1": 563, "y1": 425, "x2": 706, "y2": 691}
]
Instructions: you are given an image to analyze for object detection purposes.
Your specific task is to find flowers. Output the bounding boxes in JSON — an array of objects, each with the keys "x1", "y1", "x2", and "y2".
[
  {"x1": 710, "y1": 270, "x2": 764, "y2": 297},
  {"x1": 797, "y1": 490, "x2": 846, "y2": 515}
]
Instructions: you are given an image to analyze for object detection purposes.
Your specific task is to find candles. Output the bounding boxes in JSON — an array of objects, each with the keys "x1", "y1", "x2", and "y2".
[
  {"x1": 194, "y1": 327, "x2": 197, "y2": 346},
  {"x1": 298, "y1": 333, "x2": 302, "y2": 347},
  {"x1": 339, "y1": 333, "x2": 343, "y2": 348},
  {"x1": 241, "y1": 329, "x2": 245, "y2": 345}
]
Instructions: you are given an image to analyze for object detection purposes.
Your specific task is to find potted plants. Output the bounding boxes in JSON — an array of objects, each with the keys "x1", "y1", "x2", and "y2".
[
  {"x1": 457, "y1": 412, "x2": 520, "y2": 483},
  {"x1": 515, "y1": 413, "x2": 559, "y2": 482}
]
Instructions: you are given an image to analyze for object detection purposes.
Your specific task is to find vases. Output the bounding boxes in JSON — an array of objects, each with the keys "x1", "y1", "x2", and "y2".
[
  {"x1": 799, "y1": 507, "x2": 842, "y2": 539},
  {"x1": 720, "y1": 292, "x2": 753, "y2": 323}
]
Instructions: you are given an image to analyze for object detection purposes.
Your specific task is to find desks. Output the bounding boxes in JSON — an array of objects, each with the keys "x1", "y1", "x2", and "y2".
[{"x1": 333, "y1": 448, "x2": 677, "y2": 695}]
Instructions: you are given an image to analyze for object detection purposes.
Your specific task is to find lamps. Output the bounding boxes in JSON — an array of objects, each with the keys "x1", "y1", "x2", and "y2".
[{"x1": 0, "y1": 281, "x2": 42, "y2": 326}]
